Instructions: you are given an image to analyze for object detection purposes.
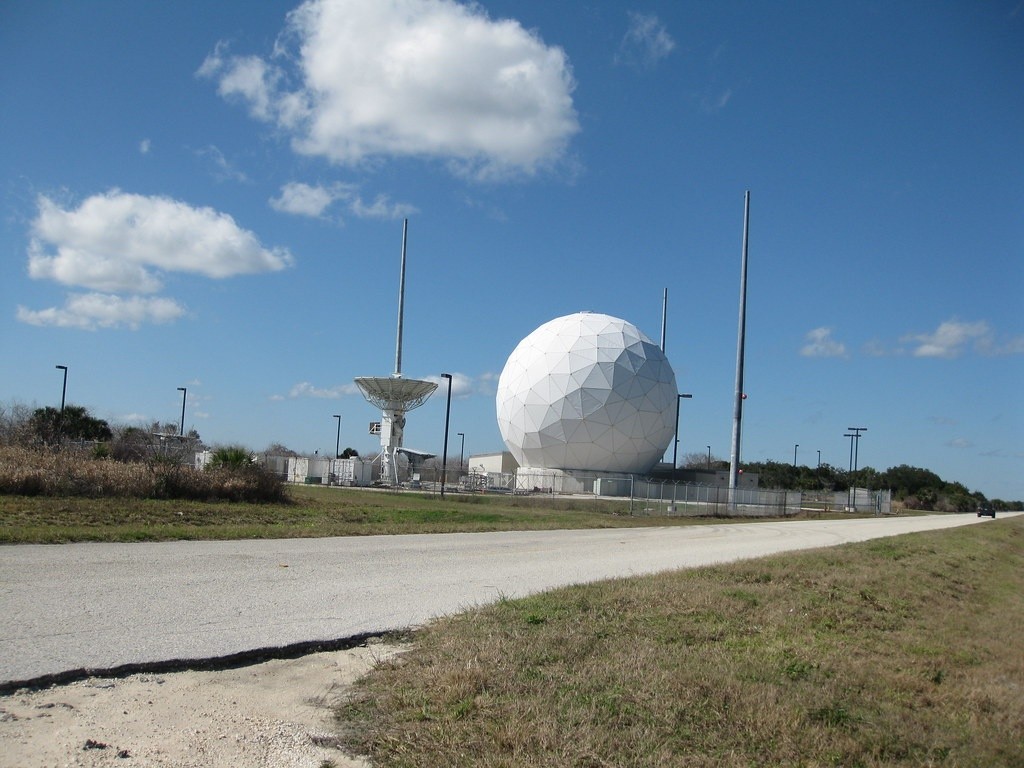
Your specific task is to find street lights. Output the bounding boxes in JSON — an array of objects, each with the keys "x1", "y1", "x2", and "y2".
[
  {"x1": 843, "y1": 434, "x2": 862, "y2": 506},
  {"x1": 707, "y1": 445, "x2": 710, "y2": 458},
  {"x1": 177, "y1": 387, "x2": 186, "y2": 435},
  {"x1": 440, "y1": 374, "x2": 452, "y2": 496},
  {"x1": 848, "y1": 427, "x2": 867, "y2": 507},
  {"x1": 794, "y1": 445, "x2": 799, "y2": 466},
  {"x1": 673, "y1": 394, "x2": 692, "y2": 474},
  {"x1": 332, "y1": 415, "x2": 341, "y2": 459},
  {"x1": 55, "y1": 365, "x2": 67, "y2": 420},
  {"x1": 817, "y1": 451, "x2": 820, "y2": 467},
  {"x1": 457, "y1": 433, "x2": 464, "y2": 471}
]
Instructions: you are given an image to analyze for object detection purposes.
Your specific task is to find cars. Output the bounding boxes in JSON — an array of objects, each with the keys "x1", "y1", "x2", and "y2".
[{"x1": 978, "y1": 503, "x2": 995, "y2": 518}]
